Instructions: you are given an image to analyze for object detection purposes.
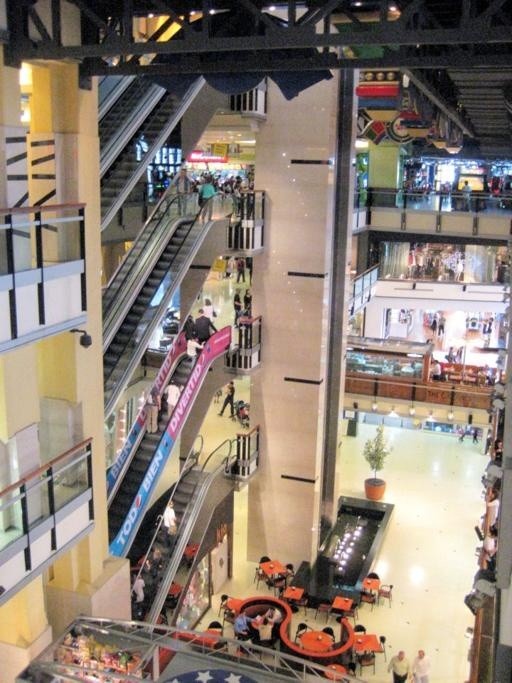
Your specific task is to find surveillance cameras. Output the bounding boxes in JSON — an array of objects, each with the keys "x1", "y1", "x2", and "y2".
[{"x1": 80, "y1": 335, "x2": 93, "y2": 349}]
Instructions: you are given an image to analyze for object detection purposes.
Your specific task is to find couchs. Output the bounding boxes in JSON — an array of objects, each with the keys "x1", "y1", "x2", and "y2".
[{"x1": 162, "y1": 317, "x2": 178, "y2": 334}]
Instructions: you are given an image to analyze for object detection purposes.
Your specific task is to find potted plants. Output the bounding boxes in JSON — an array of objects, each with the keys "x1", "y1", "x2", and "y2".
[{"x1": 364, "y1": 424, "x2": 392, "y2": 500}]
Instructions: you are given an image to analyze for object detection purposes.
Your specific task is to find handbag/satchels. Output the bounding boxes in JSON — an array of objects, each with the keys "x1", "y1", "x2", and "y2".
[{"x1": 198, "y1": 191, "x2": 203, "y2": 205}]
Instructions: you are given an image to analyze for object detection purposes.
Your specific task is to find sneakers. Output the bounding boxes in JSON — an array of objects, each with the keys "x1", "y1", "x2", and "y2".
[{"x1": 218, "y1": 413, "x2": 234, "y2": 418}]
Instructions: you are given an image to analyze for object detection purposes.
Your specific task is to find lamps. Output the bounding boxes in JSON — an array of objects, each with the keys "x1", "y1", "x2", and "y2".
[
  {"x1": 400, "y1": 119, "x2": 462, "y2": 154},
  {"x1": 464, "y1": 593, "x2": 479, "y2": 615}
]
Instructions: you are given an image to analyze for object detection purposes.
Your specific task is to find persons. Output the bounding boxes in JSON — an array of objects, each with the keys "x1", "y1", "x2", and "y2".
[
  {"x1": 430, "y1": 312, "x2": 438, "y2": 335},
  {"x1": 387, "y1": 649, "x2": 413, "y2": 683},
  {"x1": 472, "y1": 430, "x2": 479, "y2": 444},
  {"x1": 438, "y1": 312, "x2": 447, "y2": 336},
  {"x1": 165, "y1": 378, "x2": 183, "y2": 419},
  {"x1": 129, "y1": 496, "x2": 182, "y2": 616},
  {"x1": 448, "y1": 346, "x2": 457, "y2": 364},
  {"x1": 412, "y1": 649, "x2": 432, "y2": 683},
  {"x1": 481, "y1": 437, "x2": 503, "y2": 564},
  {"x1": 431, "y1": 359, "x2": 441, "y2": 381},
  {"x1": 141, "y1": 385, "x2": 163, "y2": 435},
  {"x1": 217, "y1": 380, "x2": 236, "y2": 419},
  {"x1": 176, "y1": 168, "x2": 244, "y2": 225},
  {"x1": 460, "y1": 180, "x2": 472, "y2": 213},
  {"x1": 177, "y1": 254, "x2": 254, "y2": 370},
  {"x1": 232, "y1": 605, "x2": 284, "y2": 654},
  {"x1": 456, "y1": 346, "x2": 463, "y2": 364}
]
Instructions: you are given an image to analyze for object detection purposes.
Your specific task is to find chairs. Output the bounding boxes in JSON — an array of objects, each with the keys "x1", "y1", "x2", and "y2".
[
  {"x1": 209, "y1": 556, "x2": 393, "y2": 682},
  {"x1": 439, "y1": 362, "x2": 506, "y2": 386}
]
